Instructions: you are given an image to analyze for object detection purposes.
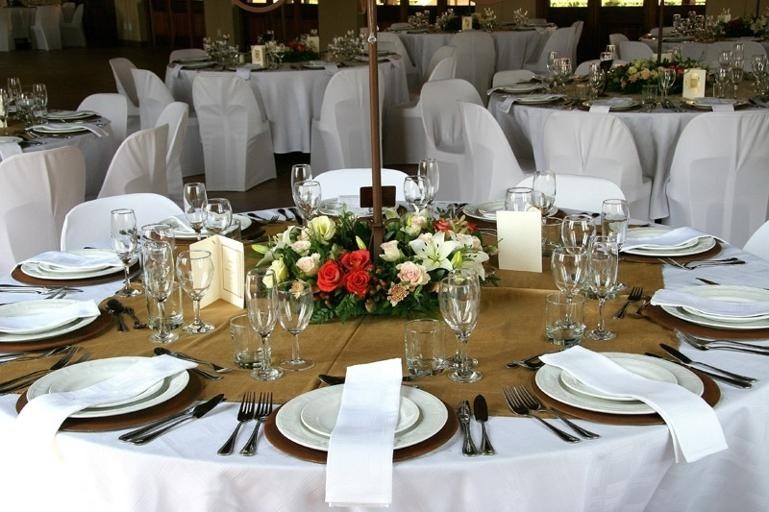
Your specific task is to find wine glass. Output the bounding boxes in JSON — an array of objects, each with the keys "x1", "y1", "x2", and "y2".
[
  {"x1": 202, "y1": 28, "x2": 367, "y2": 66},
  {"x1": 439, "y1": 277, "x2": 484, "y2": 382},
  {"x1": 104, "y1": 202, "x2": 315, "y2": 384},
  {"x1": 673, "y1": 8, "x2": 731, "y2": 41},
  {"x1": 0, "y1": 75, "x2": 50, "y2": 127},
  {"x1": 409, "y1": 8, "x2": 529, "y2": 32},
  {"x1": 586, "y1": 236, "x2": 619, "y2": 342},
  {"x1": 551, "y1": 246, "x2": 589, "y2": 331},
  {"x1": 544, "y1": 44, "x2": 769, "y2": 105},
  {"x1": 600, "y1": 198, "x2": 630, "y2": 291},
  {"x1": 446, "y1": 269, "x2": 481, "y2": 368},
  {"x1": 560, "y1": 214, "x2": 597, "y2": 292}
]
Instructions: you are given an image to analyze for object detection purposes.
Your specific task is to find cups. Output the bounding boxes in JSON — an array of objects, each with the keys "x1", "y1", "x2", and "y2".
[
  {"x1": 531, "y1": 167, "x2": 556, "y2": 214},
  {"x1": 418, "y1": 159, "x2": 441, "y2": 208},
  {"x1": 404, "y1": 318, "x2": 444, "y2": 378},
  {"x1": 143, "y1": 277, "x2": 272, "y2": 368},
  {"x1": 504, "y1": 186, "x2": 535, "y2": 211},
  {"x1": 545, "y1": 294, "x2": 585, "y2": 348},
  {"x1": 403, "y1": 174, "x2": 428, "y2": 211},
  {"x1": 182, "y1": 155, "x2": 321, "y2": 238}
]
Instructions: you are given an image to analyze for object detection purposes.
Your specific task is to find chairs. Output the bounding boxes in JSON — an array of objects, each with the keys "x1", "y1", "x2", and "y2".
[{"x1": 2, "y1": 92, "x2": 190, "y2": 268}]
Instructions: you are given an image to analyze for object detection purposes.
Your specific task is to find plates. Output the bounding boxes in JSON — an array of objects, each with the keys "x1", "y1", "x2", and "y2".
[
  {"x1": 533, "y1": 350, "x2": 704, "y2": 418},
  {"x1": 28, "y1": 109, "x2": 98, "y2": 135},
  {"x1": 274, "y1": 381, "x2": 450, "y2": 452},
  {"x1": 660, "y1": 284, "x2": 769, "y2": 330},
  {"x1": 496, "y1": 80, "x2": 750, "y2": 113},
  {"x1": 462, "y1": 200, "x2": 558, "y2": 222},
  {"x1": 226, "y1": 47, "x2": 394, "y2": 70},
  {"x1": 25, "y1": 355, "x2": 191, "y2": 418},
  {"x1": 1, "y1": 249, "x2": 132, "y2": 345},
  {"x1": 618, "y1": 225, "x2": 717, "y2": 256}
]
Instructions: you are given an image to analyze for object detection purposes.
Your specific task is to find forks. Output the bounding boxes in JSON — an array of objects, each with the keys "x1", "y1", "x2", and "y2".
[
  {"x1": 666, "y1": 326, "x2": 769, "y2": 363},
  {"x1": 611, "y1": 285, "x2": 657, "y2": 320},
  {"x1": 1, "y1": 345, "x2": 81, "y2": 395},
  {"x1": 657, "y1": 255, "x2": 747, "y2": 271},
  {"x1": 496, "y1": 383, "x2": 596, "y2": 446},
  {"x1": 217, "y1": 390, "x2": 274, "y2": 458}
]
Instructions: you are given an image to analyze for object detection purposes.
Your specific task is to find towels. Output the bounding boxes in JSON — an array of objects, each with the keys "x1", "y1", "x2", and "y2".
[
  {"x1": 7, "y1": 352, "x2": 199, "y2": 462},
  {"x1": 649, "y1": 286, "x2": 766, "y2": 321},
  {"x1": 535, "y1": 342, "x2": 730, "y2": 468},
  {"x1": 323, "y1": 353, "x2": 407, "y2": 508},
  {"x1": 0, "y1": 299, "x2": 102, "y2": 331}
]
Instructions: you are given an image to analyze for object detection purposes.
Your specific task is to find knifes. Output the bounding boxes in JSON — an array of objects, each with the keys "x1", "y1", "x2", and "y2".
[
  {"x1": 119, "y1": 392, "x2": 224, "y2": 447},
  {"x1": 456, "y1": 391, "x2": 498, "y2": 457},
  {"x1": 645, "y1": 343, "x2": 759, "y2": 390}
]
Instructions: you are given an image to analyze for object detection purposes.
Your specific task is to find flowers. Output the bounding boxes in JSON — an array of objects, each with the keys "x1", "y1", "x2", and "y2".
[{"x1": 256, "y1": 205, "x2": 500, "y2": 323}]
